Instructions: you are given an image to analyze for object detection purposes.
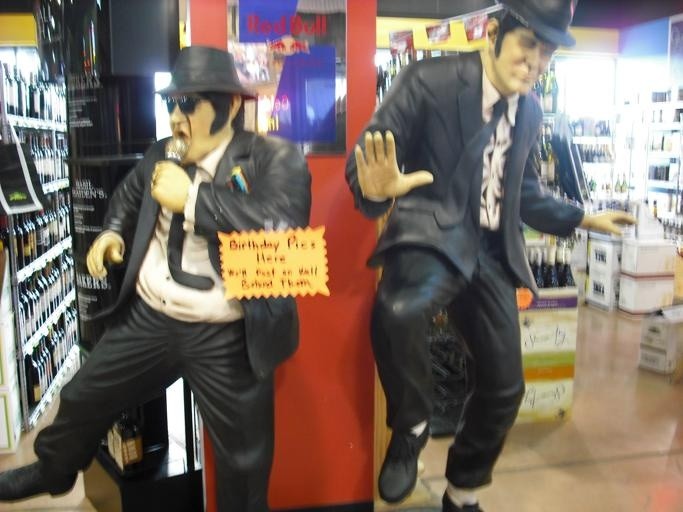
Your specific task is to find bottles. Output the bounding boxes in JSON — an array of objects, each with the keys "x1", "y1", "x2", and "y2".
[
  {"x1": 528, "y1": 248, "x2": 574, "y2": 287},
  {"x1": 21, "y1": 304, "x2": 90, "y2": 405},
  {"x1": 651, "y1": 201, "x2": 683, "y2": 241},
  {"x1": 14, "y1": 249, "x2": 85, "y2": 347},
  {"x1": 538, "y1": 73, "x2": 553, "y2": 113},
  {"x1": 614, "y1": 172, "x2": 628, "y2": 193},
  {"x1": 378, "y1": 48, "x2": 411, "y2": 101},
  {"x1": 540, "y1": 127, "x2": 555, "y2": 181},
  {"x1": 27, "y1": 133, "x2": 70, "y2": 185},
  {"x1": 579, "y1": 145, "x2": 611, "y2": 161},
  {"x1": 3, "y1": 65, "x2": 64, "y2": 120},
  {"x1": 108, "y1": 413, "x2": 145, "y2": 472},
  {"x1": 8, "y1": 193, "x2": 73, "y2": 271}
]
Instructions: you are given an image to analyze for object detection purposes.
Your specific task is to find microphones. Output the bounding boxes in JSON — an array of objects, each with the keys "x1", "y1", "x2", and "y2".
[{"x1": 163, "y1": 136, "x2": 188, "y2": 164}]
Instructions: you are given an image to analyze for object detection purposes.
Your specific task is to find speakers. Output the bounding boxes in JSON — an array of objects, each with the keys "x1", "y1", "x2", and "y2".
[{"x1": 96, "y1": 0, "x2": 181, "y2": 81}]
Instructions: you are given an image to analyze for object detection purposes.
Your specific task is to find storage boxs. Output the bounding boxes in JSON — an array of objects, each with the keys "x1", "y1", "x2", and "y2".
[
  {"x1": 616, "y1": 270, "x2": 676, "y2": 315},
  {"x1": 565, "y1": 231, "x2": 628, "y2": 313},
  {"x1": 620, "y1": 240, "x2": 678, "y2": 280},
  {"x1": 638, "y1": 305, "x2": 683, "y2": 377}
]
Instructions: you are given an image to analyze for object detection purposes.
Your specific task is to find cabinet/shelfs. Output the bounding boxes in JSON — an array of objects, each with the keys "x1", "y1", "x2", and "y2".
[
  {"x1": 0, "y1": 63, "x2": 78, "y2": 433},
  {"x1": 521, "y1": 242, "x2": 579, "y2": 415},
  {"x1": 519, "y1": 96, "x2": 682, "y2": 246}
]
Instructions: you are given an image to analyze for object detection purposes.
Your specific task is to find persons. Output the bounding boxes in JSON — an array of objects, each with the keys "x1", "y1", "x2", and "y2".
[
  {"x1": 345, "y1": 0, "x2": 637, "y2": 512},
  {"x1": 0, "y1": 47, "x2": 313, "y2": 512}
]
